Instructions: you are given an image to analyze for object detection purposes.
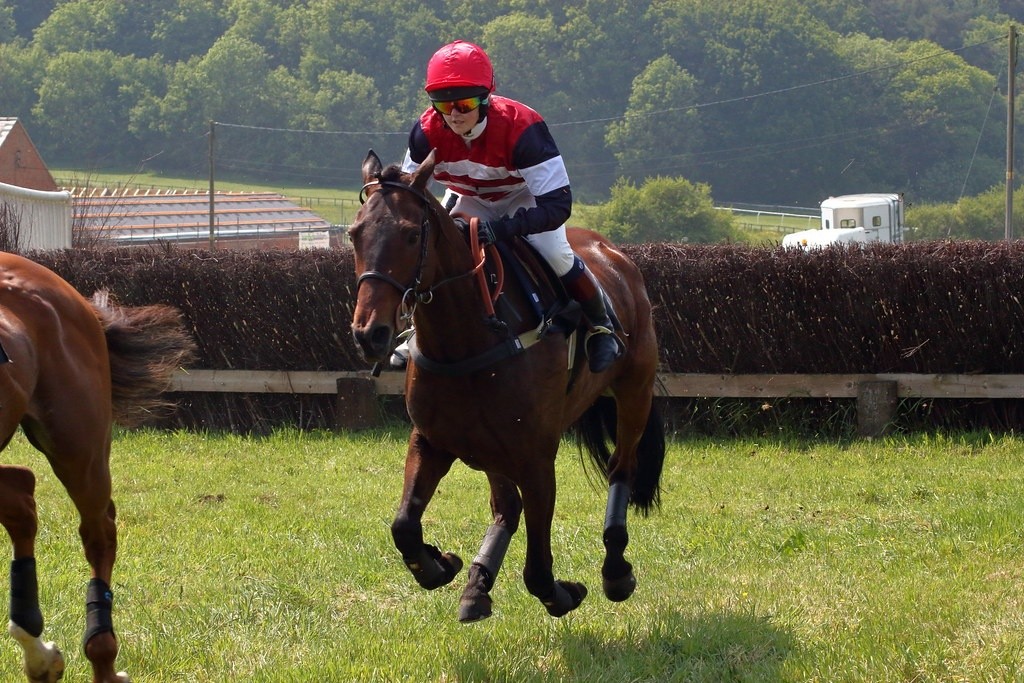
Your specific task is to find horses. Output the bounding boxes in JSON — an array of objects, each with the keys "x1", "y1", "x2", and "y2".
[
  {"x1": 0, "y1": 251, "x2": 200, "y2": 683},
  {"x1": 347, "y1": 148, "x2": 668, "y2": 625}
]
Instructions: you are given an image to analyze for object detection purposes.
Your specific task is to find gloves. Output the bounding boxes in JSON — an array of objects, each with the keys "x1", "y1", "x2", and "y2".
[{"x1": 463, "y1": 220, "x2": 504, "y2": 246}]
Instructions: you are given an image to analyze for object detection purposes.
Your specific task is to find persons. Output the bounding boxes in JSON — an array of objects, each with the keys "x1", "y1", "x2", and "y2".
[{"x1": 401, "y1": 39, "x2": 622, "y2": 374}]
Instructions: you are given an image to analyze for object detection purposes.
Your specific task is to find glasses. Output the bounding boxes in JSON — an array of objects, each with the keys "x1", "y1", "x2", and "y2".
[{"x1": 430, "y1": 95, "x2": 484, "y2": 115}]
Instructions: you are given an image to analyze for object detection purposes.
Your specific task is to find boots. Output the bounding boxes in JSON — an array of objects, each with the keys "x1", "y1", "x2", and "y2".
[{"x1": 557, "y1": 256, "x2": 620, "y2": 373}]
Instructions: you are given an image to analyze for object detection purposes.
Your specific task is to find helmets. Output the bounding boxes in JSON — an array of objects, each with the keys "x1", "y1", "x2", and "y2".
[{"x1": 423, "y1": 40, "x2": 495, "y2": 101}]
